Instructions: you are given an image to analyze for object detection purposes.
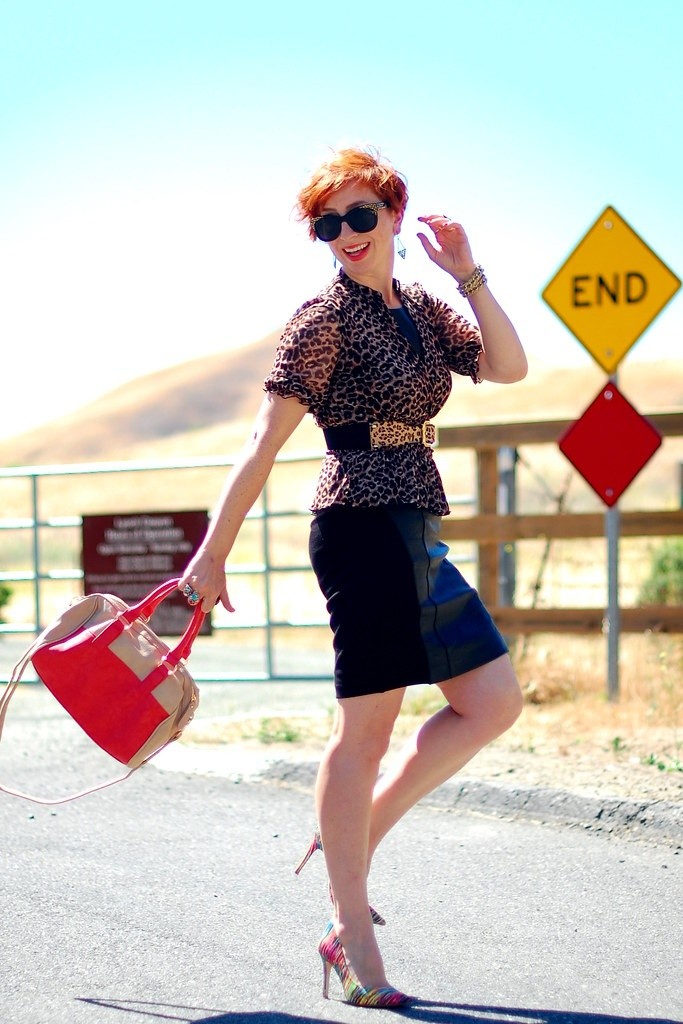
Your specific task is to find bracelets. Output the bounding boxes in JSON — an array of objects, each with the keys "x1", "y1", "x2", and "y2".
[{"x1": 456, "y1": 264, "x2": 488, "y2": 298}]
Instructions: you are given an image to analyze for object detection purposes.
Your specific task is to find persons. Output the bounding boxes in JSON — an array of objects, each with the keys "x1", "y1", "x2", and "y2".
[{"x1": 178, "y1": 148, "x2": 525, "y2": 1007}]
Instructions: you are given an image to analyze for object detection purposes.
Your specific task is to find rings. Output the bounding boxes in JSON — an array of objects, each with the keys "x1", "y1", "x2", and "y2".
[
  {"x1": 183, "y1": 584, "x2": 193, "y2": 594},
  {"x1": 189, "y1": 594, "x2": 199, "y2": 604},
  {"x1": 435, "y1": 229, "x2": 440, "y2": 234},
  {"x1": 443, "y1": 215, "x2": 453, "y2": 221}
]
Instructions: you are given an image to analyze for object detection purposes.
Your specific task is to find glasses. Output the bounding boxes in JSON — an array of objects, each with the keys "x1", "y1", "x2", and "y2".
[{"x1": 310, "y1": 201, "x2": 389, "y2": 242}]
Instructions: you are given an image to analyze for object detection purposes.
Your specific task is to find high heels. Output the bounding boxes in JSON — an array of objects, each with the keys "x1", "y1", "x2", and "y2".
[
  {"x1": 296, "y1": 825, "x2": 386, "y2": 926},
  {"x1": 318, "y1": 922, "x2": 416, "y2": 1008}
]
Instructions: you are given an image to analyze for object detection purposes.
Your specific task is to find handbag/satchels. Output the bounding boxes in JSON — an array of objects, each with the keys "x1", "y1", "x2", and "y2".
[{"x1": 29, "y1": 577, "x2": 208, "y2": 770}]
